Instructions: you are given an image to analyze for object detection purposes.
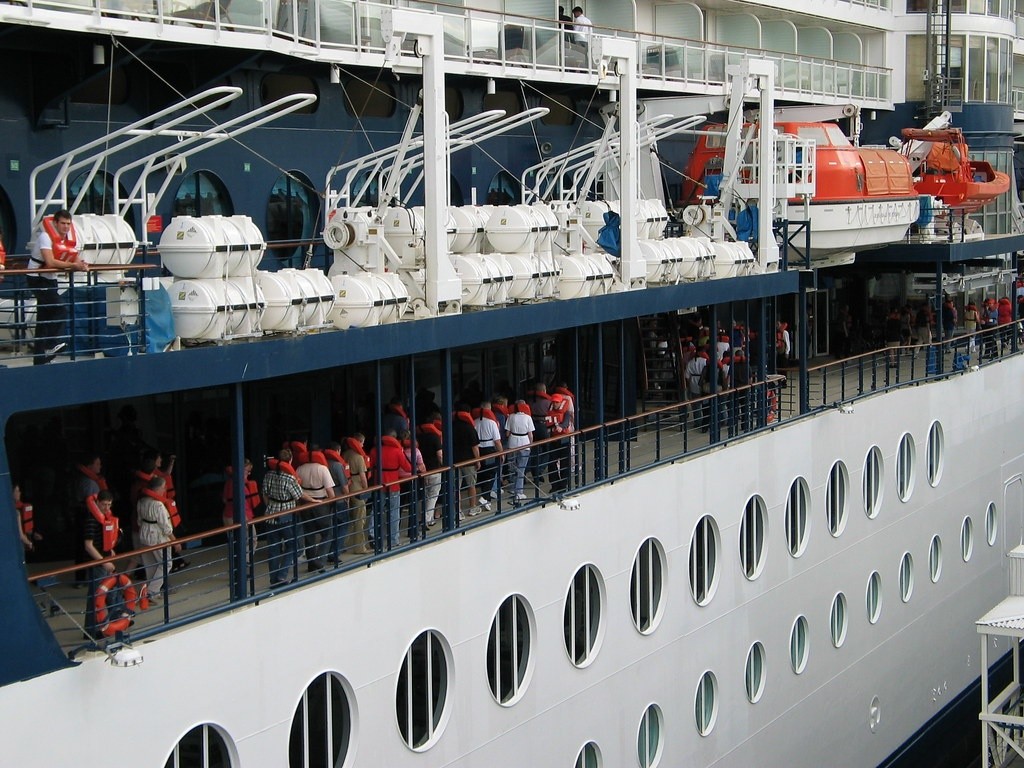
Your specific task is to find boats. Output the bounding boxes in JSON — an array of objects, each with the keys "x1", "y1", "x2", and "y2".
[
  {"x1": 683, "y1": 117, "x2": 922, "y2": 264},
  {"x1": 900, "y1": 126, "x2": 1011, "y2": 214}
]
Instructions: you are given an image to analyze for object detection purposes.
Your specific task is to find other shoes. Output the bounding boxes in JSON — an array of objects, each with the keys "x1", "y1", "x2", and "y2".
[
  {"x1": 172, "y1": 561, "x2": 193, "y2": 572},
  {"x1": 501, "y1": 489, "x2": 505, "y2": 494},
  {"x1": 145, "y1": 594, "x2": 157, "y2": 607},
  {"x1": 419, "y1": 520, "x2": 430, "y2": 531},
  {"x1": 352, "y1": 548, "x2": 375, "y2": 554},
  {"x1": 491, "y1": 492, "x2": 497, "y2": 499},
  {"x1": 368, "y1": 534, "x2": 376, "y2": 549},
  {"x1": 83, "y1": 630, "x2": 105, "y2": 641},
  {"x1": 270, "y1": 579, "x2": 289, "y2": 589},
  {"x1": 72, "y1": 579, "x2": 84, "y2": 588},
  {"x1": 478, "y1": 497, "x2": 491, "y2": 510},
  {"x1": 327, "y1": 556, "x2": 342, "y2": 565},
  {"x1": 429, "y1": 521, "x2": 436, "y2": 526},
  {"x1": 516, "y1": 493, "x2": 527, "y2": 500},
  {"x1": 44, "y1": 343, "x2": 66, "y2": 357}
]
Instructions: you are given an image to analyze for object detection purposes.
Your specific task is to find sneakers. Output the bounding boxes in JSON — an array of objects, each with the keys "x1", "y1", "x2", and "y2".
[
  {"x1": 469, "y1": 506, "x2": 482, "y2": 516},
  {"x1": 459, "y1": 511, "x2": 466, "y2": 520}
]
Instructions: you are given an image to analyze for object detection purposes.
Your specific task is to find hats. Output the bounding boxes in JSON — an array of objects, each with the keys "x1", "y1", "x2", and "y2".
[{"x1": 551, "y1": 394, "x2": 563, "y2": 403}]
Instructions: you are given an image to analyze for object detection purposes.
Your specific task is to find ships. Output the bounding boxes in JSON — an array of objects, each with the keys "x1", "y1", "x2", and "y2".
[{"x1": 3, "y1": 0, "x2": 1024, "y2": 768}]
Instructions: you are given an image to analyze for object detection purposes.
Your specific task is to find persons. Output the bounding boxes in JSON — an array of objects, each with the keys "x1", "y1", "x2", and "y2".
[
  {"x1": 0, "y1": 235, "x2": 6, "y2": 280},
  {"x1": 647, "y1": 318, "x2": 790, "y2": 433},
  {"x1": 233, "y1": 381, "x2": 577, "y2": 604},
  {"x1": 12, "y1": 484, "x2": 41, "y2": 548},
  {"x1": 559, "y1": 6, "x2": 573, "y2": 30},
  {"x1": 76, "y1": 454, "x2": 190, "y2": 588},
  {"x1": 886, "y1": 274, "x2": 1024, "y2": 368},
  {"x1": 572, "y1": 6, "x2": 592, "y2": 73},
  {"x1": 84, "y1": 489, "x2": 134, "y2": 640},
  {"x1": 138, "y1": 478, "x2": 183, "y2": 603},
  {"x1": 27, "y1": 210, "x2": 89, "y2": 366}
]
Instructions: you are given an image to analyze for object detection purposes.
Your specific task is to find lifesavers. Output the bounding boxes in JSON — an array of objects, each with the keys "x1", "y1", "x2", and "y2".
[
  {"x1": 766, "y1": 391, "x2": 778, "y2": 423},
  {"x1": 96, "y1": 573, "x2": 137, "y2": 637}
]
